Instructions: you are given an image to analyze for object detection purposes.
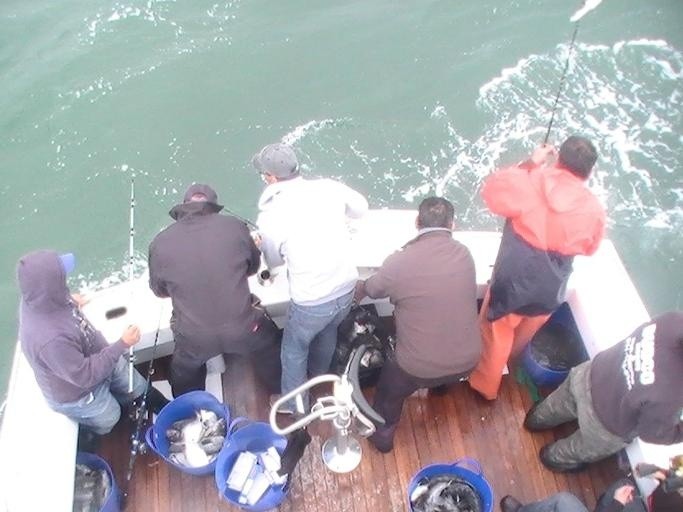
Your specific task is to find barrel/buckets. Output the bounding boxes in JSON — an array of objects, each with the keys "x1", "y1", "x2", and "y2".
[
  {"x1": 146, "y1": 390, "x2": 231, "y2": 478},
  {"x1": 522, "y1": 318, "x2": 587, "y2": 390},
  {"x1": 74, "y1": 452, "x2": 123, "y2": 512},
  {"x1": 408, "y1": 456, "x2": 496, "y2": 512},
  {"x1": 215, "y1": 416, "x2": 294, "y2": 512}
]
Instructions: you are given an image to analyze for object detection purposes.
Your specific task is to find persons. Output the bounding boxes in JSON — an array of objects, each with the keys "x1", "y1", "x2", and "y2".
[
  {"x1": 524, "y1": 312, "x2": 683, "y2": 472},
  {"x1": 17, "y1": 250, "x2": 171, "y2": 438},
  {"x1": 355, "y1": 198, "x2": 481, "y2": 453},
  {"x1": 467, "y1": 135, "x2": 603, "y2": 400},
  {"x1": 499, "y1": 471, "x2": 683, "y2": 511},
  {"x1": 147, "y1": 183, "x2": 280, "y2": 398},
  {"x1": 253, "y1": 142, "x2": 369, "y2": 415}
]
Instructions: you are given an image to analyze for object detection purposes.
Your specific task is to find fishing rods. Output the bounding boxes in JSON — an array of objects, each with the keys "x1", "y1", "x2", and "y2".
[
  {"x1": 544, "y1": 24, "x2": 579, "y2": 150},
  {"x1": 120, "y1": 328, "x2": 160, "y2": 509},
  {"x1": 128, "y1": 178, "x2": 137, "y2": 393},
  {"x1": 221, "y1": 208, "x2": 260, "y2": 235}
]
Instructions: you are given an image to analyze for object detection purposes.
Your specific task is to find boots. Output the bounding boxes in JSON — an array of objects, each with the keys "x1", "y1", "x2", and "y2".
[
  {"x1": 132, "y1": 381, "x2": 171, "y2": 415},
  {"x1": 106, "y1": 404, "x2": 153, "y2": 440}
]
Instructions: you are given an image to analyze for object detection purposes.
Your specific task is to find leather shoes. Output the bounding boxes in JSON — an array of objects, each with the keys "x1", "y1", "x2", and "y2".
[{"x1": 500, "y1": 494, "x2": 523, "y2": 512}]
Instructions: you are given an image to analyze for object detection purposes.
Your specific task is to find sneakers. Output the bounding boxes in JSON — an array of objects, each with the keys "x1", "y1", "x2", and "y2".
[
  {"x1": 352, "y1": 414, "x2": 392, "y2": 452},
  {"x1": 270, "y1": 393, "x2": 295, "y2": 414}
]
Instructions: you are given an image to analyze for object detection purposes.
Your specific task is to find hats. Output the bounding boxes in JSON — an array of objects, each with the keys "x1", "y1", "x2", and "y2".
[
  {"x1": 183, "y1": 183, "x2": 218, "y2": 204},
  {"x1": 60, "y1": 253, "x2": 76, "y2": 273},
  {"x1": 252, "y1": 143, "x2": 299, "y2": 178}
]
